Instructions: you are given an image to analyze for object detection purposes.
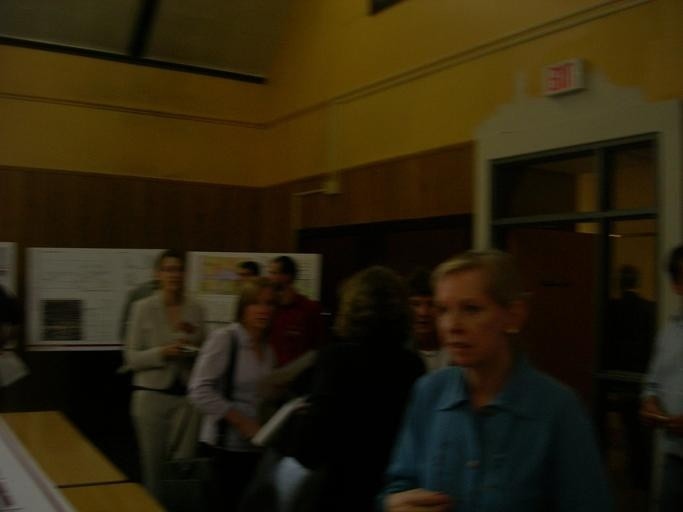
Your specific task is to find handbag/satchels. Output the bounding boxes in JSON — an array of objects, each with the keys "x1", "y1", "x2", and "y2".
[{"x1": 180, "y1": 441, "x2": 229, "y2": 499}]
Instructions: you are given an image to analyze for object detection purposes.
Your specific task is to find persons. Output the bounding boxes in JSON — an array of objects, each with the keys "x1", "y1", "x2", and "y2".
[{"x1": 125, "y1": 247, "x2": 682, "y2": 512}]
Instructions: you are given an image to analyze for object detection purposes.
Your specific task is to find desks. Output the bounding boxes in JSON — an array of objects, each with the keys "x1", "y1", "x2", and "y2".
[{"x1": 0, "y1": 411, "x2": 165, "y2": 511}]
